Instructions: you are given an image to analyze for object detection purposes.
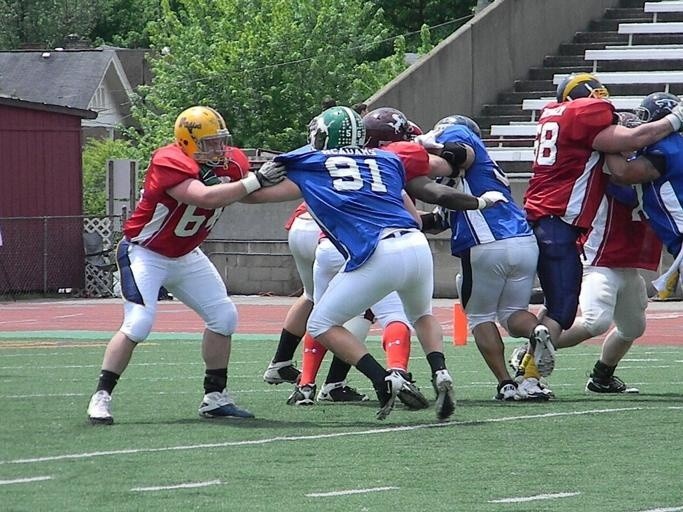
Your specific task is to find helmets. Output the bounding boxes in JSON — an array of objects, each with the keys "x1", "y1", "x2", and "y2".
[
  {"x1": 308, "y1": 105, "x2": 368, "y2": 151},
  {"x1": 433, "y1": 113, "x2": 483, "y2": 140},
  {"x1": 639, "y1": 90, "x2": 680, "y2": 124},
  {"x1": 611, "y1": 109, "x2": 642, "y2": 130},
  {"x1": 172, "y1": 103, "x2": 231, "y2": 162},
  {"x1": 361, "y1": 105, "x2": 410, "y2": 149},
  {"x1": 555, "y1": 72, "x2": 608, "y2": 102}
]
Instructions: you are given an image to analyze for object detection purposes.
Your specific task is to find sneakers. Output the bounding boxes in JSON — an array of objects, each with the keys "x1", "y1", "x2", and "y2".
[
  {"x1": 85, "y1": 388, "x2": 115, "y2": 428},
  {"x1": 280, "y1": 382, "x2": 317, "y2": 409},
  {"x1": 262, "y1": 357, "x2": 301, "y2": 385},
  {"x1": 528, "y1": 322, "x2": 557, "y2": 378},
  {"x1": 316, "y1": 378, "x2": 369, "y2": 405},
  {"x1": 198, "y1": 388, "x2": 258, "y2": 421},
  {"x1": 584, "y1": 367, "x2": 642, "y2": 397},
  {"x1": 432, "y1": 368, "x2": 457, "y2": 422},
  {"x1": 374, "y1": 371, "x2": 402, "y2": 422},
  {"x1": 493, "y1": 342, "x2": 558, "y2": 405},
  {"x1": 398, "y1": 371, "x2": 428, "y2": 410}
]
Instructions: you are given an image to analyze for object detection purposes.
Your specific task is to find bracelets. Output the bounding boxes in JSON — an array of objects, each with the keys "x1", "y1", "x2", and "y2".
[{"x1": 240, "y1": 174, "x2": 261, "y2": 195}]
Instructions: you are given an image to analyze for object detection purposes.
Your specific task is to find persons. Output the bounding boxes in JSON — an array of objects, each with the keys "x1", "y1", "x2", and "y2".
[{"x1": 86, "y1": 107, "x2": 289, "y2": 422}]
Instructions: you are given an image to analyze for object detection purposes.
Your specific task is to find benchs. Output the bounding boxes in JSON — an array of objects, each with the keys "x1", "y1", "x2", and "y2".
[{"x1": 476, "y1": 1, "x2": 682, "y2": 182}]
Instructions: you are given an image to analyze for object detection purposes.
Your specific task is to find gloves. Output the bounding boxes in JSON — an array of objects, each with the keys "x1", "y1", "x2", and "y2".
[
  {"x1": 198, "y1": 163, "x2": 223, "y2": 186},
  {"x1": 241, "y1": 158, "x2": 290, "y2": 195},
  {"x1": 415, "y1": 126, "x2": 445, "y2": 150},
  {"x1": 475, "y1": 189, "x2": 511, "y2": 212}
]
